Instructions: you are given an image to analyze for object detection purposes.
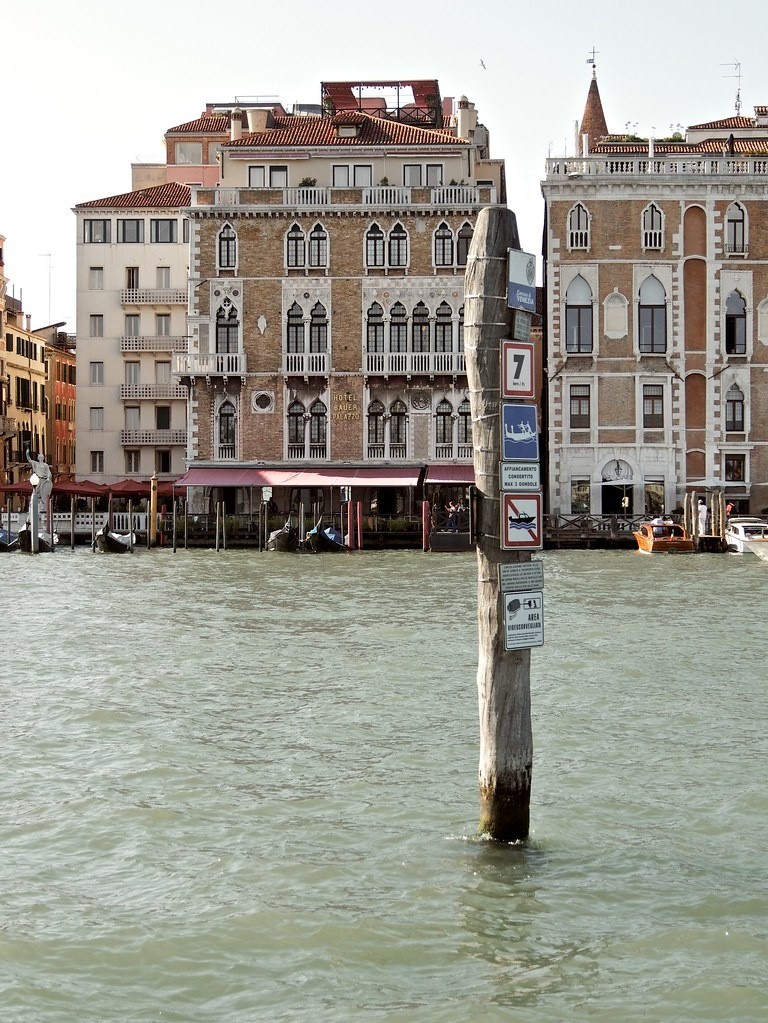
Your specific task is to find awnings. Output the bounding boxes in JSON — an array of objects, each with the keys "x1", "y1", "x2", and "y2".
[
  {"x1": 172, "y1": 468, "x2": 422, "y2": 487},
  {"x1": 424, "y1": 464, "x2": 476, "y2": 483}
]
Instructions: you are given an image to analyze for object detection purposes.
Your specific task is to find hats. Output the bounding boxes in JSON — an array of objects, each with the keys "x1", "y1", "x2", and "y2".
[{"x1": 698, "y1": 500, "x2": 703, "y2": 503}]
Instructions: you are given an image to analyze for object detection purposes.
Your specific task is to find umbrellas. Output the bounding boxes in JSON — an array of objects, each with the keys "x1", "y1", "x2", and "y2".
[
  {"x1": 675, "y1": 476, "x2": 748, "y2": 495},
  {"x1": 591, "y1": 477, "x2": 656, "y2": 519}
]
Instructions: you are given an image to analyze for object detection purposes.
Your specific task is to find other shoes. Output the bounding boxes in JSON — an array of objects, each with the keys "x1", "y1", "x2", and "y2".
[
  {"x1": 441, "y1": 529, "x2": 444, "y2": 532},
  {"x1": 451, "y1": 530, "x2": 456, "y2": 532}
]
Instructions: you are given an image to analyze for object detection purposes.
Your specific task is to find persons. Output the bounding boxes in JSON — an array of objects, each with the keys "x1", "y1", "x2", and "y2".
[
  {"x1": 697, "y1": 499, "x2": 707, "y2": 536},
  {"x1": 441, "y1": 498, "x2": 468, "y2": 533},
  {"x1": 25, "y1": 449, "x2": 53, "y2": 509},
  {"x1": 651, "y1": 514, "x2": 665, "y2": 537}
]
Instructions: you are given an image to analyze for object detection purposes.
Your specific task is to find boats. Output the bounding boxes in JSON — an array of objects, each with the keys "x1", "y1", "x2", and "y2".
[
  {"x1": 0, "y1": 526, "x2": 20, "y2": 552},
  {"x1": 306, "y1": 514, "x2": 348, "y2": 552},
  {"x1": 724, "y1": 517, "x2": 768, "y2": 553},
  {"x1": 632, "y1": 517, "x2": 696, "y2": 555},
  {"x1": 17, "y1": 516, "x2": 60, "y2": 553},
  {"x1": 95, "y1": 521, "x2": 136, "y2": 552},
  {"x1": 267, "y1": 513, "x2": 299, "y2": 552}
]
